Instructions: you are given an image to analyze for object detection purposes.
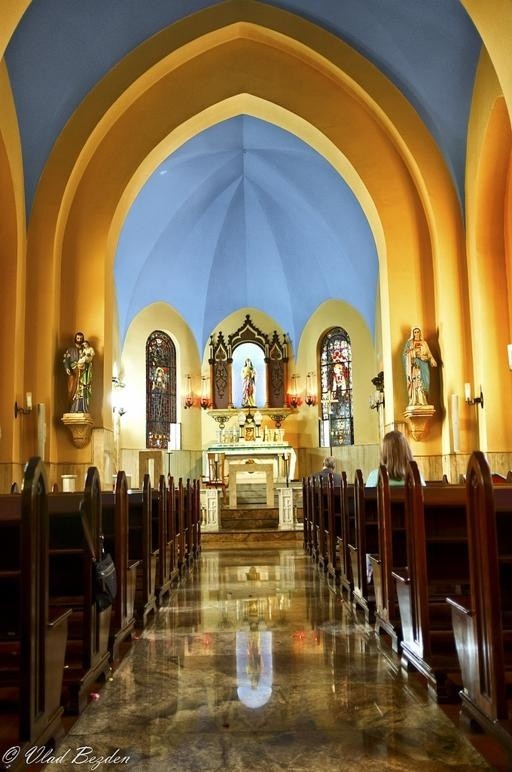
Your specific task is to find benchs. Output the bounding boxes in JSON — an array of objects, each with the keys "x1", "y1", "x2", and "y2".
[
  {"x1": 1, "y1": 453, "x2": 201, "y2": 771},
  {"x1": 301, "y1": 451, "x2": 511, "y2": 772}
]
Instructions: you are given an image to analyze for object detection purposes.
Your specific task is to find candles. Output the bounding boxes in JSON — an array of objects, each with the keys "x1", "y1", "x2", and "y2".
[
  {"x1": 284, "y1": 452, "x2": 287, "y2": 460},
  {"x1": 26, "y1": 392, "x2": 32, "y2": 412},
  {"x1": 167, "y1": 442, "x2": 170, "y2": 452},
  {"x1": 465, "y1": 382, "x2": 469, "y2": 400},
  {"x1": 214, "y1": 454, "x2": 217, "y2": 463}
]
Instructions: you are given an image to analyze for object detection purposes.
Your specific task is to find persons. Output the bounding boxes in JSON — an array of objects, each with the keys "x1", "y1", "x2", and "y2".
[
  {"x1": 62, "y1": 333, "x2": 95, "y2": 413},
  {"x1": 365, "y1": 431, "x2": 426, "y2": 487},
  {"x1": 328, "y1": 361, "x2": 349, "y2": 396},
  {"x1": 402, "y1": 326, "x2": 438, "y2": 405},
  {"x1": 242, "y1": 358, "x2": 256, "y2": 407},
  {"x1": 151, "y1": 367, "x2": 170, "y2": 392},
  {"x1": 311, "y1": 456, "x2": 342, "y2": 487}
]
